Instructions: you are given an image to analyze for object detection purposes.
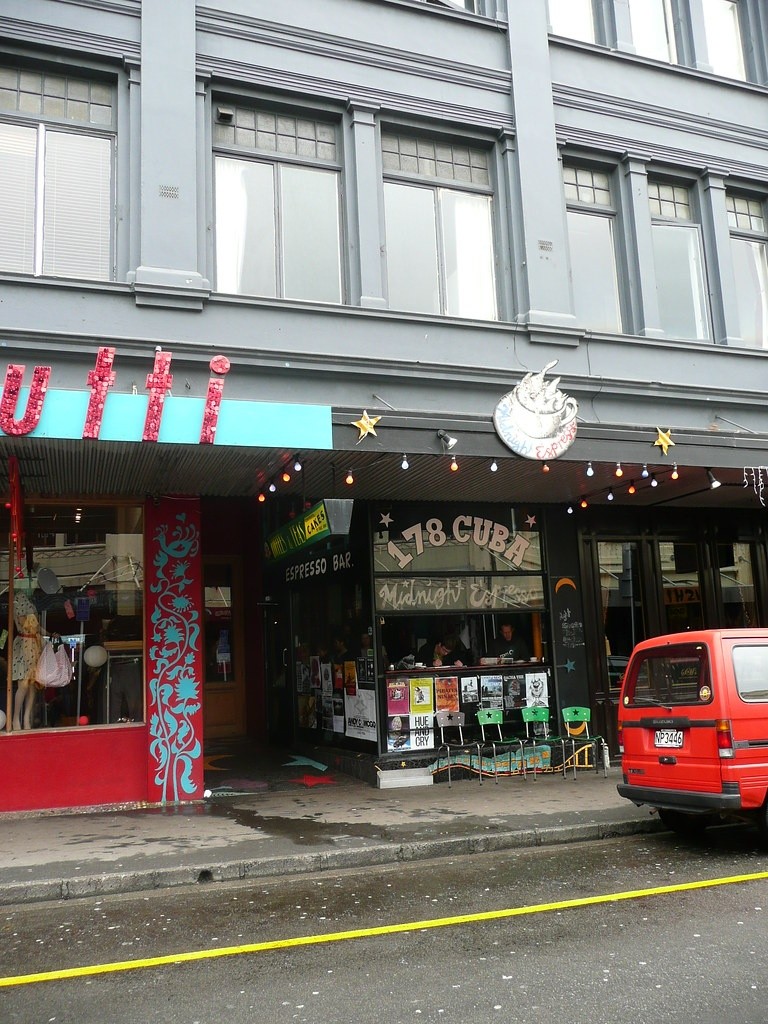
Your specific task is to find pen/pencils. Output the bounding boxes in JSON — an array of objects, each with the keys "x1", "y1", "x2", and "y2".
[{"x1": 436, "y1": 654, "x2": 442, "y2": 666}]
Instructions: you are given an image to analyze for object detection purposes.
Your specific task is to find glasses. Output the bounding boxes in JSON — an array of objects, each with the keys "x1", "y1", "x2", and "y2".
[{"x1": 440, "y1": 647, "x2": 447, "y2": 653}]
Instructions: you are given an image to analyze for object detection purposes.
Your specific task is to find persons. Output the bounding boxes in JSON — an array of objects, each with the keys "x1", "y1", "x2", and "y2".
[
  {"x1": 13, "y1": 574, "x2": 62, "y2": 730},
  {"x1": 315, "y1": 633, "x2": 373, "y2": 664},
  {"x1": 479, "y1": 623, "x2": 530, "y2": 666},
  {"x1": 416, "y1": 634, "x2": 466, "y2": 667}
]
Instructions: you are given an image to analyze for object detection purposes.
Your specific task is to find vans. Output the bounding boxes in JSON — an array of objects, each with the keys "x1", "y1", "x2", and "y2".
[{"x1": 614, "y1": 626, "x2": 768, "y2": 836}]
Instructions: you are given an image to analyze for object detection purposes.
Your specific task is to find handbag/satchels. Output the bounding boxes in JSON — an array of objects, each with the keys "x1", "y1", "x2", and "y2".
[{"x1": 35, "y1": 633, "x2": 72, "y2": 688}]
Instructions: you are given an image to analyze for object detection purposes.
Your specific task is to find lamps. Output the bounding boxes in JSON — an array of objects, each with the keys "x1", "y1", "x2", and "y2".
[
  {"x1": 705, "y1": 469, "x2": 721, "y2": 490},
  {"x1": 437, "y1": 429, "x2": 459, "y2": 450}
]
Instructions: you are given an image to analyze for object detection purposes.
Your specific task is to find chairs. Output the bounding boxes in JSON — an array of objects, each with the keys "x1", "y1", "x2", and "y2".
[
  {"x1": 435, "y1": 713, "x2": 482, "y2": 788},
  {"x1": 520, "y1": 706, "x2": 567, "y2": 782},
  {"x1": 476, "y1": 709, "x2": 527, "y2": 785},
  {"x1": 561, "y1": 706, "x2": 606, "y2": 781}
]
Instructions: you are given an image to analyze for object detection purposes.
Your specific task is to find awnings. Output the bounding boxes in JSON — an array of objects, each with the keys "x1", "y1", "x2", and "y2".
[{"x1": 601, "y1": 573, "x2": 755, "y2": 606}]
